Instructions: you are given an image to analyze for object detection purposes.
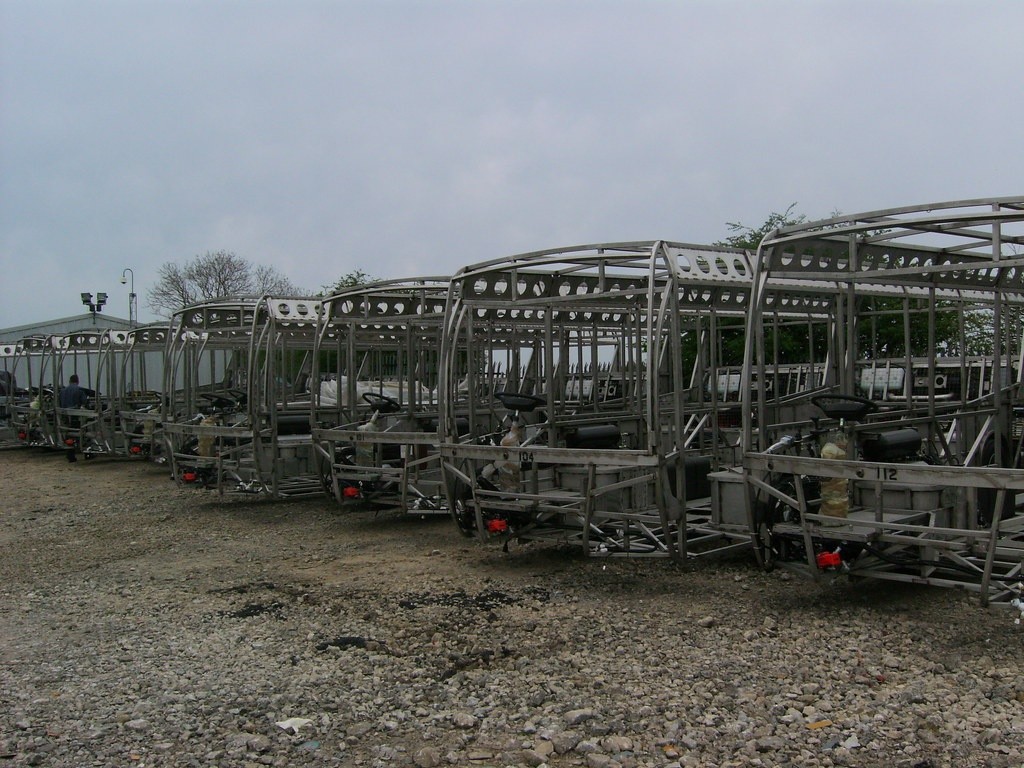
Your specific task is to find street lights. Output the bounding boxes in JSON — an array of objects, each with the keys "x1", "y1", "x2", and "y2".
[{"x1": 121, "y1": 268, "x2": 138, "y2": 327}]
